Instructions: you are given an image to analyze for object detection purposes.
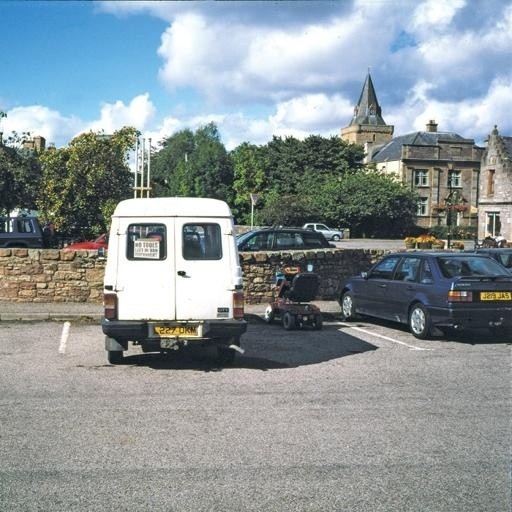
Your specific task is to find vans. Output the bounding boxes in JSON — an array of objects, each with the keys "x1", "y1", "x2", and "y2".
[{"x1": 96, "y1": 193, "x2": 249, "y2": 365}]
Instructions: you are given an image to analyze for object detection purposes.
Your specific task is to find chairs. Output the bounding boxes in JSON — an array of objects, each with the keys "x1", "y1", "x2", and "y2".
[
  {"x1": 405, "y1": 266, "x2": 428, "y2": 283},
  {"x1": 147, "y1": 232, "x2": 164, "y2": 259},
  {"x1": 183, "y1": 232, "x2": 203, "y2": 260},
  {"x1": 284, "y1": 273, "x2": 321, "y2": 303}
]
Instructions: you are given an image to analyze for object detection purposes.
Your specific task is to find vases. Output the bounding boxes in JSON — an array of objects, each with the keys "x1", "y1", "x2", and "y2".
[{"x1": 406, "y1": 242, "x2": 463, "y2": 250}]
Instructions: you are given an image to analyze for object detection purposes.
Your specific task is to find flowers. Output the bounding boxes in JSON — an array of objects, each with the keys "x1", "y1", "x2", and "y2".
[{"x1": 404, "y1": 234, "x2": 465, "y2": 247}]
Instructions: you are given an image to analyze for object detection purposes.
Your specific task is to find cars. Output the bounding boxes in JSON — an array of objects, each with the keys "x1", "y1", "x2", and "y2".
[
  {"x1": 64, "y1": 232, "x2": 109, "y2": 250},
  {"x1": 302, "y1": 222, "x2": 344, "y2": 242},
  {"x1": 336, "y1": 251, "x2": 512, "y2": 341},
  {"x1": 461, "y1": 247, "x2": 511, "y2": 277}
]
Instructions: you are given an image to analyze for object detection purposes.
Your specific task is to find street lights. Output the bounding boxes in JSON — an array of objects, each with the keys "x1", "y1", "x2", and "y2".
[{"x1": 444, "y1": 156, "x2": 457, "y2": 249}]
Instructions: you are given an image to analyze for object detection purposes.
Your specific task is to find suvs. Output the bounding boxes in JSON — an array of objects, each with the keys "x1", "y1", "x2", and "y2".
[
  {"x1": 235, "y1": 225, "x2": 336, "y2": 253},
  {"x1": 0, "y1": 215, "x2": 47, "y2": 248}
]
